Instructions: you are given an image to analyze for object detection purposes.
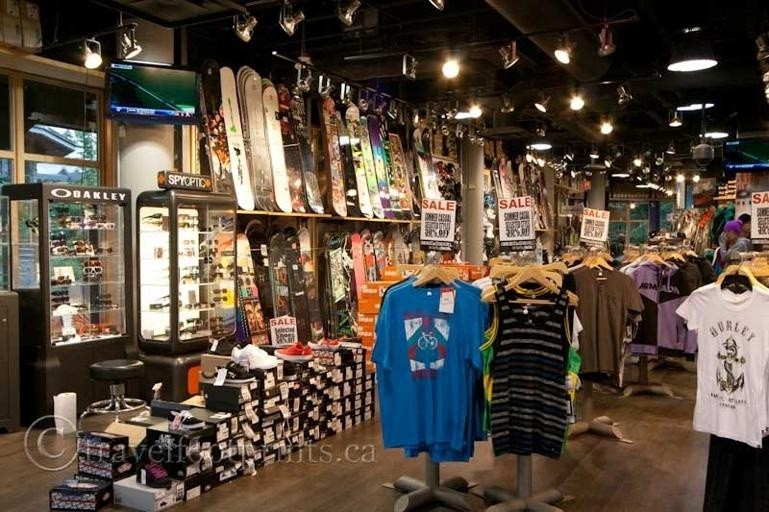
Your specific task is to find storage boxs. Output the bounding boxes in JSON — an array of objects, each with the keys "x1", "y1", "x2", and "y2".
[{"x1": 49, "y1": 268, "x2": 487, "y2": 512}]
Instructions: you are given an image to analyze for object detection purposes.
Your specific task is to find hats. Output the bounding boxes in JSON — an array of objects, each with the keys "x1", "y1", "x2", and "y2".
[{"x1": 724, "y1": 217, "x2": 744, "y2": 234}]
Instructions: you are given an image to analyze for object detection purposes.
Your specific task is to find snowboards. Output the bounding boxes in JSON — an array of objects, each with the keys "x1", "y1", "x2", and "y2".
[
  {"x1": 491, "y1": 158, "x2": 550, "y2": 231},
  {"x1": 237, "y1": 219, "x2": 409, "y2": 348},
  {"x1": 196, "y1": 58, "x2": 440, "y2": 219}
]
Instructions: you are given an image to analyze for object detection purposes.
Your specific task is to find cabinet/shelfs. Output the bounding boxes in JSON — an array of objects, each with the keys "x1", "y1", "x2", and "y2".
[
  {"x1": 1, "y1": 182, "x2": 138, "y2": 427},
  {"x1": 135, "y1": 188, "x2": 237, "y2": 352}
]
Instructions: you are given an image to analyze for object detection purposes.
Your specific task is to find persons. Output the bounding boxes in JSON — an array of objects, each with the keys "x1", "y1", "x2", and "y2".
[
  {"x1": 719, "y1": 219, "x2": 751, "y2": 265},
  {"x1": 737, "y1": 212, "x2": 751, "y2": 240}
]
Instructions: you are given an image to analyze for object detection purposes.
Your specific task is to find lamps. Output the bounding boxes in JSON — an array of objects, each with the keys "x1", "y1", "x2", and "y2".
[
  {"x1": 118, "y1": 31, "x2": 143, "y2": 61},
  {"x1": 81, "y1": 40, "x2": 103, "y2": 69},
  {"x1": 231, "y1": 1, "x2": 744, "y2": 169}
]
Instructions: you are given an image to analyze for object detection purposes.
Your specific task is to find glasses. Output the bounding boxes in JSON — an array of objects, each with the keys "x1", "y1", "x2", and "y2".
[{"x1": 25, "y1": 204, "x2": 236, "y2": 344}]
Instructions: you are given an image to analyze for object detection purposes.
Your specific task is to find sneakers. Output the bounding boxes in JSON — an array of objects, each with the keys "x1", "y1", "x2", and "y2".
[
  {"x1": 308, "y1": 337, "x2": 339, "y2": 350},
  {"x1": 136, "y1": 462, "x2": 171, "y2": 488},
  {"x1": 214, "y1": 361, "x2": 256, "y2": 383},
  {"x1": 274, "y1": 342, "x2": 313, "y2": 361},
  {"x1": 167, "y1": 410, "x2": 204, "y2": 429},
  {"x1": 231, "y1": 343, "x2": 277, "y2": 370},
  {"x1": 338, "y1": 337, "x2": 362, "y2": 347}
]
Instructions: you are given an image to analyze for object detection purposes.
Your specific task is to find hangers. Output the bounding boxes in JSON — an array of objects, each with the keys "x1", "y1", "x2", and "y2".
[{"x1": 408, "y1": 237, "x2": 769, "y2": 305}]
